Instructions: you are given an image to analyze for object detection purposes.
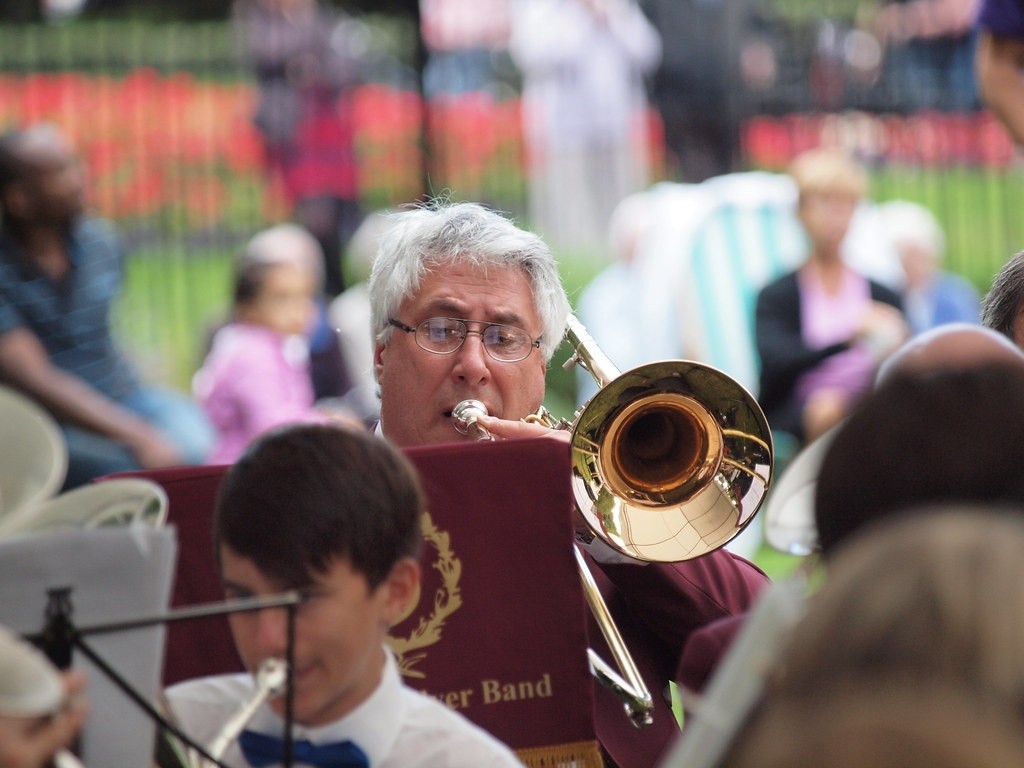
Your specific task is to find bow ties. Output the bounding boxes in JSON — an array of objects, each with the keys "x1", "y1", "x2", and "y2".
[{"x1": 235, "y1": 729, "x2": 371, "y2": 768}]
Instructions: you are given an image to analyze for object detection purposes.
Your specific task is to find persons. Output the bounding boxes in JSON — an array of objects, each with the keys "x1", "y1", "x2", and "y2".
[
  {"x1": 193, "y1": 208, "x2": 398, "y2": 464},
  {"x1": 0, "y1": 122, "x2": 218, "y2": 479},
  {"x1": 729, "y1": 253, "x2": 1024, "y2": 768},
  {"x1": 579, "y1": 157, "x2": 984, "y2": 475},
  {"x1": 164, "y1": 421, "x2": 525, "y2": 768},
  {"x1": 369, "y1": 201, "x2": 771, "y2": 768}
]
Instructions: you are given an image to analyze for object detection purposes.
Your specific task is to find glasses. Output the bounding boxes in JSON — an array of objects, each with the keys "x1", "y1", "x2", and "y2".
[{"x1": 387, "y1": 316, "x2": 548, "y2": 363}]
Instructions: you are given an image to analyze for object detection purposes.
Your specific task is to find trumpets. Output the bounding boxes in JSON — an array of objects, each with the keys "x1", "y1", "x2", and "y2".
[{"x1": 187, "y1": 656, "x2": 288, "y2": 768}]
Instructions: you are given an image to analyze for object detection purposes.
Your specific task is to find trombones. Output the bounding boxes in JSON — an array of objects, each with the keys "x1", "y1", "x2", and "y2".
[{"x1": 450, "y1": 312, "x2": 778, "y2": 729}]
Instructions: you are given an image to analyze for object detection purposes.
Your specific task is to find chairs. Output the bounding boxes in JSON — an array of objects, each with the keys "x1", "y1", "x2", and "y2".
[
  {"x1": 673, "y1": 176, "x2": 904, "y2": 463},
  {"x1": 0, "y1": 389, "x2": 168, "y2": 528}
]
